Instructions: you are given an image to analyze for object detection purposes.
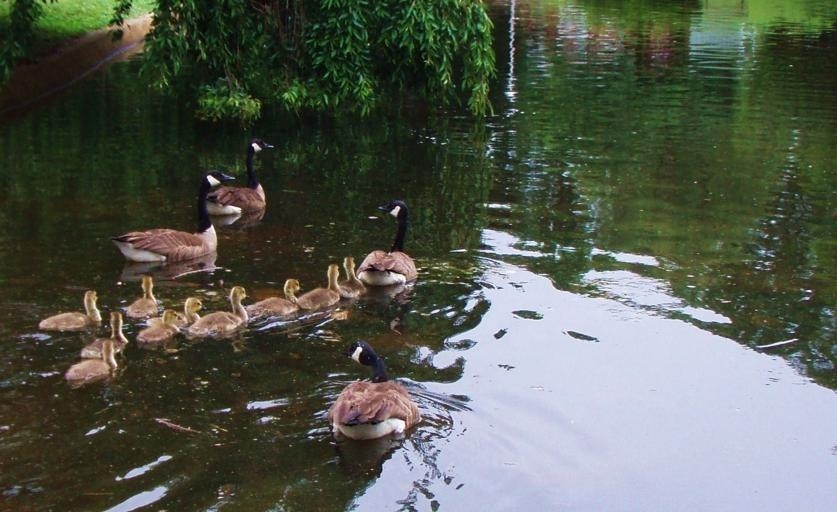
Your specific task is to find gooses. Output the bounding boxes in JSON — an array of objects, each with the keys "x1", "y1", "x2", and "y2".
[
  {"x1": 322, "y1": 339, "x2": 421, "y2": 441},
  {"x1": 208, "y1": 137, "x2": 274, "y2": 216},
  {"x1": 136, "y1": 308, "x2": 182, "y2": 343},
  {"x1": 247, "y1": 278, "x2": 299, "y2": 315},
  {"x1": 183, "y1": 285, "x2": 249, "y2": 334},
  {"x1": 110, "y1": 169, "x2": 238, "y2": 264},
  {"x1": 145, "y1": 297, "x2": 208, "y2": 325},
  {"x1": 339, "y1": 255, "x2": 367, "y2": 300},
  {"x1": 299, "y1": 263, "x2": 342, "y2": 310},
  {"x1": 38, "y1": 288, "x2": 104, "y2": 332},
  {"x1": 64, "y1": 340, "x2": 119, "y2": 384},
  {"x1": 356, "y1": 198, "x2": 418, "y2": 285},
  {"x1": 126, "y1": 275, "x2": 159, "y2": 318}
]
